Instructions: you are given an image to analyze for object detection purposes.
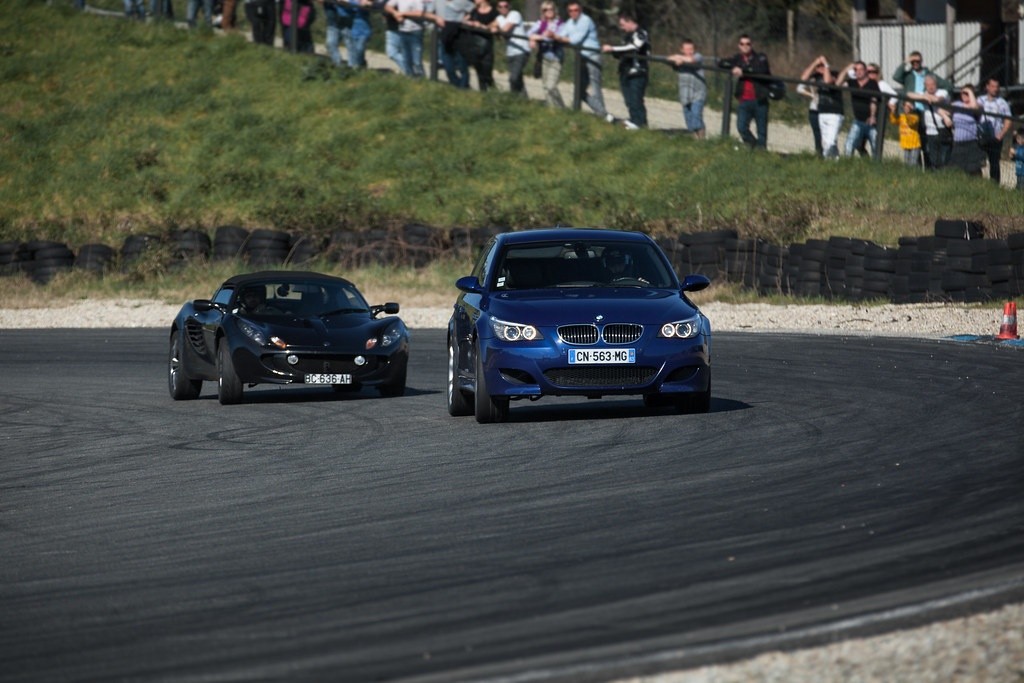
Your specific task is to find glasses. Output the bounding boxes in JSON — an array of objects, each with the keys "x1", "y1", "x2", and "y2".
[
  {"x1": 498, "y1": 5, "x2": 509, "y2": 9},
  {"x1": 568, "y1": 8, "x2": 579, "y2": 12},
  {"x1": 739, "y1": 42, "x2": 752, "y2": 46},
  {"x1": 867, "y1": 70, "x2": 877, "y2": 74}
]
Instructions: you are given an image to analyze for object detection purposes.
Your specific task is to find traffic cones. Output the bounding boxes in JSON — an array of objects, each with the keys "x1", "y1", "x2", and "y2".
[{"x1": 995, "y1": 301, "x2": 1020, "y2": 340}]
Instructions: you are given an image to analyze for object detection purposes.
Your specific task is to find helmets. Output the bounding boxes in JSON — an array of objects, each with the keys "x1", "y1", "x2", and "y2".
[{"x1": 601, "y1": 246, "x2": 634, "y2": 268}]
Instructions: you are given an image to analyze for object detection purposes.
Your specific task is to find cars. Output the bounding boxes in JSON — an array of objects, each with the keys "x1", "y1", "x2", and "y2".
[
  {"x1": 443, "y1": 227, "x2": 716, "y2": 427},
  {"x1": 166, "y1": 269, "x2": 412, "y2": 407}
]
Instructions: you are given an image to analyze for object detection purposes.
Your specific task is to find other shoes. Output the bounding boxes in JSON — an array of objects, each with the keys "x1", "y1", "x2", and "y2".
[
  {"x1": 623, "y1": 119, "x2": 639, "y2": 130},
  {"x1": 605, "y1": 113, "x2": 615, "y2": 122}
]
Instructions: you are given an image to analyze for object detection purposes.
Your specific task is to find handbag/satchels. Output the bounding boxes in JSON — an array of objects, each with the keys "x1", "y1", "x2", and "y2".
[
  {"x1": 938, "y1": 128, "x2": 954, "y2": 145},
  {"x1": 534, "y1": 51, "x2": 541, "y2": 78},
  {"x1": 977, "y1": 105, "x2": 996, "y2": 148},
  {"x1": 767, "y1": 81, "x2": 787, "y2": 100}
]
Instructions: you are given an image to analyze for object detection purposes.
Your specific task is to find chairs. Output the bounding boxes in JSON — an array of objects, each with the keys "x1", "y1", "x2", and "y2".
[
  {"x1": 302, "y1": 287, "x2": 323, "y2": 304},
  {"x1": 501, "y1": 257, "x2": 548, "y2": 290},
  {"x1": 321, "y1": 290, "x2": 348, "y2": 312},
  {"x1": 240, "y1": 284, "x2": 267, "y2": 299}
]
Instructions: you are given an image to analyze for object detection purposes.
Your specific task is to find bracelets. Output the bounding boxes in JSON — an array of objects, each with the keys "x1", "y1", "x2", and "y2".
[{"x1": 824, "y1": 63, "x2": 829, "y2": 67}]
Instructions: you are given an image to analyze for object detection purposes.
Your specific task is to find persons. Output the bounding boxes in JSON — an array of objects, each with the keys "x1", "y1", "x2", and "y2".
[
  {"x1": 65, "y1": 0, "x2": 497, "y2": 93},
  {"x1": 601, "y1": 247, "x2": 650, "y2": 286},
  {"x1": 718, "y1": 34, "x2": 771, "y2": 149},
  {"x1": 490, "y1": 0, "x2": 531, "y2": 98},
  {"x1": 603, "y1": 10, "x2": 652, "y2": 130},
  {"x1": 1010, "y1": 127, "x2": 1024, "y2": 189},
  {"x1": 552, "y1": 0, "x2": 614, "y2": 124},
  {"x1": 666, "y1": 38, "x2": 708, "y2": 140},
  {"x1": 236, "y1": 286, "x2": 292, "y2": 315},
  {"x1": 527, "y1": 0, "x2": 565, "y2": 108},
  {"x1": 796, "y1": 51, "x2": 1012, "y2": 184}
]
